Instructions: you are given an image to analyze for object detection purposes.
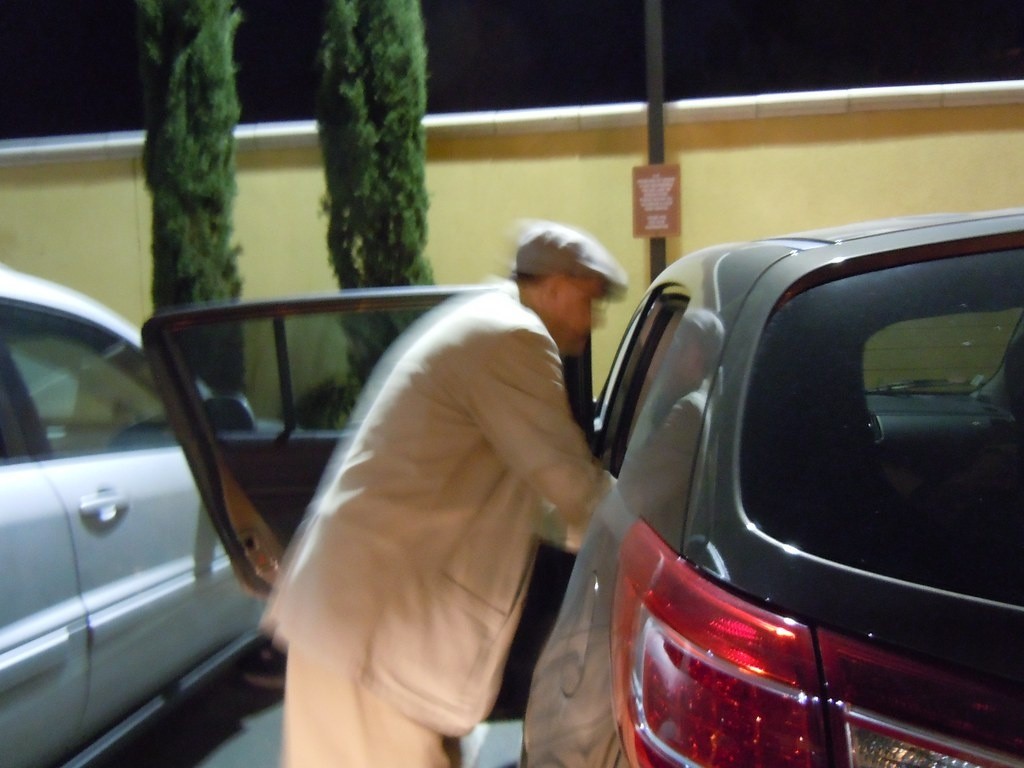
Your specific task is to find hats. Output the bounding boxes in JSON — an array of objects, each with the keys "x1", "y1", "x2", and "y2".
[{"x1": 516, "y1": 227, "x2": 627, "y2": 298}]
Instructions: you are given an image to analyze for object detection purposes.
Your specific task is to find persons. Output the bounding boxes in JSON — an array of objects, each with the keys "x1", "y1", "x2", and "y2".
[{"x1": 258, "y1": 222, "x2": 628, "y2": 768}]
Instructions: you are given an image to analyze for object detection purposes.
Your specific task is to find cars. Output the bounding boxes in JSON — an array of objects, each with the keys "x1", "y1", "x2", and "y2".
[
  {"x1": 141, "y1": 205, "x2": 1023, "y2": 768},
  {"x1": 0, "y1": 265, "x2": 286, "y2": 767}
]
